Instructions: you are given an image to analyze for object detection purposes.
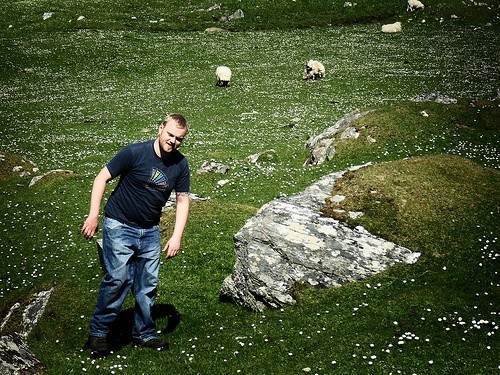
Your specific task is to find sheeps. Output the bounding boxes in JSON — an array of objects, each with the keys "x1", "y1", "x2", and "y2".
[
  {"x1": 407, "y1": 0, "x2": 425, "y2": 12},
  {"x1": 214, "y1": 65, "x2": 232, "y2": 87},
  {"x1": 303, "y1": 59, "x2": 326, "y2": 81},
  {"x1": 382, "y1": 21, "x2": 402, "y2": 33}
]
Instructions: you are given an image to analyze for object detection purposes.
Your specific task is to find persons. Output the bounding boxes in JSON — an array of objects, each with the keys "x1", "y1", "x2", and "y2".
[{"x1": 80, "y1": 112, "x2": 190, "y2": 358}]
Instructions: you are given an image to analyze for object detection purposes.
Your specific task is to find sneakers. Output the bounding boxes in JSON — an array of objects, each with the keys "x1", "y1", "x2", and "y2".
[
  {"x1": 131, "y1": 338, "x2": 169, "y2": 350},
  {"x1": 88, "y1": 334, "x2": 109, "y2": 359}
]
